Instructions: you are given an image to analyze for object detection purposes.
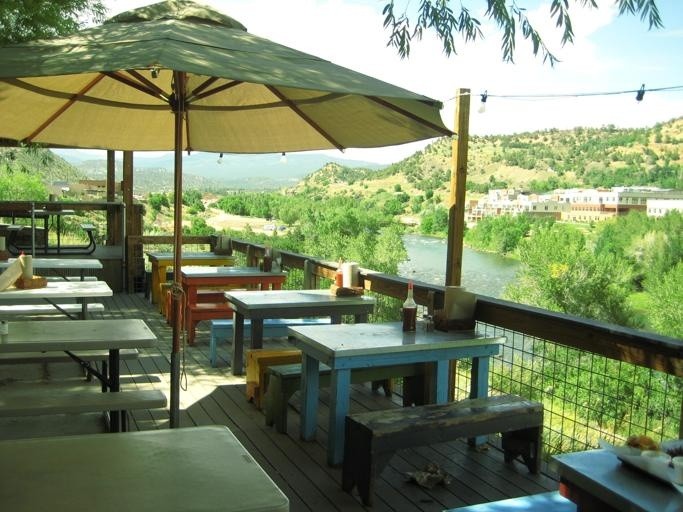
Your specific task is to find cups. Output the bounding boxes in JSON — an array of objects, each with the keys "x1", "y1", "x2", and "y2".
[{"x1": 673, "y1": 456, "x2": 683, "y2": 485}]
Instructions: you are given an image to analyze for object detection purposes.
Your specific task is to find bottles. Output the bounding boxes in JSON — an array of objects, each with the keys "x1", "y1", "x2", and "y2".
[
  {"x1": 264, "y1": 247, "x2": 273, "y2": 272},
  {"x1": 402, "y1": 280, "x2": 416, "y2": 331},
  {"x1": 336, "y1": 257, "x2": 344, "y2": 286}
]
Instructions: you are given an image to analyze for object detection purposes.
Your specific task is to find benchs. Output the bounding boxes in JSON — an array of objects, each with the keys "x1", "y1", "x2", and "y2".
[
  {"x1": 445, "y1": 491, "x2": 579, "y2": 512},
  {"x1": 166, "y1": 289, "x2": 230, "y2": 327},
  {"x1": 4, "y1": 224, "x2": 26, "y2": 256},
  {"x1": 265, "y1": 365, "x2": 435, "y2": 434},
  {"x1": 0, "y1": 389, "x2": 167, "y2": 432},
  {"x1": 0, "y1": 349, "x2": 138, "y2": 392},
  {"x1": 78, "y1": 223, "x2": 96, "y2": 255},
  {"x1": 0, "y1": 303, "x2": 104, "y2": 315},
  {"x1": 210, "y1": 318, "x2": 331, "y2": 367},
  {"x1": 45, "y1": 276, "x2": 98, "y2": 281},
  {"x1": 144, "y1": 272, "x2": 174, "y2": 300},
  {"x1": 160, "y1": 283, "x2": 174, "y2": 314},
  {"x1": 342, "y1": 396, "x2": 543, "y2": 505},
  {"x1": 247, "y1": 349, "x2": 393, "y2": 409},
  {"x1": 187, "y1": 302, "x2": 233, "y2": 346}
]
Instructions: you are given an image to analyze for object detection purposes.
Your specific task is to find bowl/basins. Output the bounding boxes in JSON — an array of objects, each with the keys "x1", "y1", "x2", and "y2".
[{"x1": 642, "y1": 450, "x2": 671, "y2": 471}]
